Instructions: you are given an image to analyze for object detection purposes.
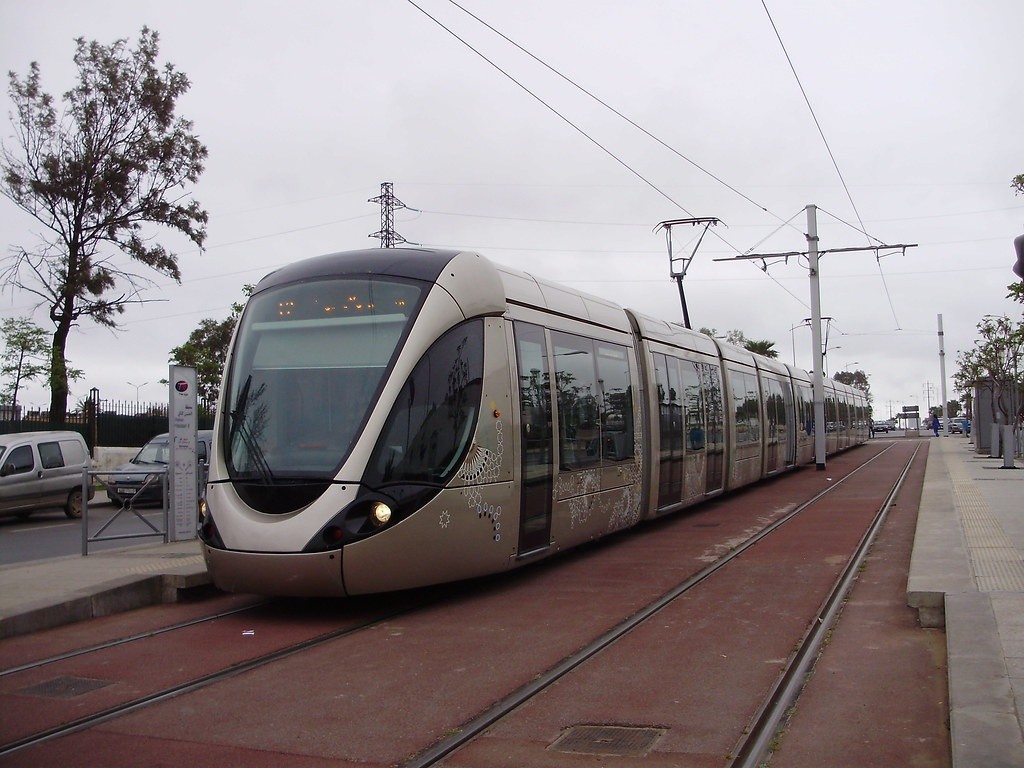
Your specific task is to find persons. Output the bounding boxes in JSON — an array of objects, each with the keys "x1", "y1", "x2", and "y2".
[
  {"x1": 868, "y1": 416, "x2": 875, "y2": 438},
  {"x1": 932, "y1": 414, "x2": 940, "y2": 437}
]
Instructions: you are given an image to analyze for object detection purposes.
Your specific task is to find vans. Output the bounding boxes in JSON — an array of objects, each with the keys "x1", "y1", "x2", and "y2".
[{"x1": 0, "y1": 430, "x2": 96, "y2": 523}]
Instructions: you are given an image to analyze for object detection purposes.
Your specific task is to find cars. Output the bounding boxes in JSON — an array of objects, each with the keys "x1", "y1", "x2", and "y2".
[
  {"x1": 925, "y1": 417, "x2": 971, "y2": 434},
  {"x1": 874, "y1": 420, "x2": 889, "y2": 434},
  {"x1": 107, "y1": 428, "x2": 220, "y2": 508},
  {"x1": 886, "y1": 420, "x2": 896, "y2": 431}
]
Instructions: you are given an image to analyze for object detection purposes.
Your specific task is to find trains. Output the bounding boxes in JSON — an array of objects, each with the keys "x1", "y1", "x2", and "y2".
[{"x1": 214, "y1": 217, "x2": 896, "y2": 638}]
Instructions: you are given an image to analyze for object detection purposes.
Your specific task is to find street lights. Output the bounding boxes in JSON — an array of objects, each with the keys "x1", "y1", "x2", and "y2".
[
  {"x1": 127, "y1": 381, "x2": 149, "y2": 402},
  {"x1": 844, "y1": 361, "x2": 859, "y2": 372},
  {"x1": 825, "y1": 345, "x2": 842, "y2": 378},
  {"x1": 789, "y1": 321, "x2": 812, "y2": 369}
]
